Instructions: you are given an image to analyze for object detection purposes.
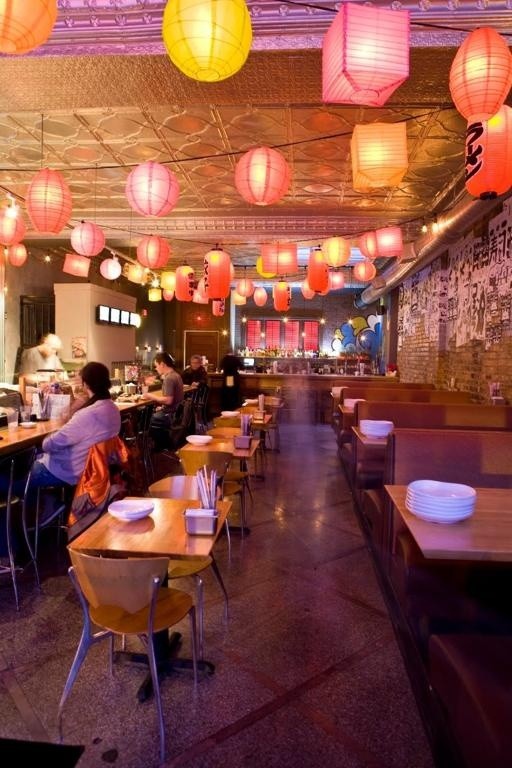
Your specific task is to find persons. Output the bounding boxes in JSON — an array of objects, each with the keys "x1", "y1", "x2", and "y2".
[
  {"x1": 144, "y1": 352, "x2": 184, "y2": 434},
  {"x1": 217, "y1": 348, "x2": 245, "y2": 408},
  {"x1": 177, "y1": 354, "x2": 206, "y2": 431},
  {"x1": 18, "y1": 361, "x2": 121, "y2": 564},
  {"x1": 20, "y1": 333, "x2": 69, "y2": 382}
]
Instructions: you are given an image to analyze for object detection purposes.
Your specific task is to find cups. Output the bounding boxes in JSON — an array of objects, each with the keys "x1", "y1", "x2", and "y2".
[
  {"x1": 199, "y1": 499, "x2": 216, "y2": 509},
  {"x1": 37, "y1": 398, "x2": 53, "y2": 420},
  {"x1": 21, "y1": 406, "x2": 30, "y2": 422},
  {"x1": 7, "y1": 411, "x2": 19, "y2": 432}
]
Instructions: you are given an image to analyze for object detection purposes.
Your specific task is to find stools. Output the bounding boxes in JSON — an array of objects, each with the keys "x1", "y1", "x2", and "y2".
[{"x1": 0, "y1": 379, "x2": 209, "y2": 615}]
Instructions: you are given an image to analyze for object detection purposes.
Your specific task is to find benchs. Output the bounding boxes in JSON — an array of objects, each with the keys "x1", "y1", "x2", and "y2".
[
  {"x1": 386, "y1": 429, "x2": 510, "y2": 635},
  {"x1": 429, "y1": 636, "x2": 512, "y2": 767},
  {"x1": 324, "y1": 378, "x2": 511, "y2": 484}
]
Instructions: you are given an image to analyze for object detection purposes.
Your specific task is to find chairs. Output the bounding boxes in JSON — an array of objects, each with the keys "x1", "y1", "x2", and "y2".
[
  {"x1": 176, "y1": 383, "x2": 282, "y2": 532},
  {"x1": 58, "y1": 547, "x2": 198, "y2": 761},
  {"x1": 125, "y1": 475, "x2": 230, "y2": 647}
]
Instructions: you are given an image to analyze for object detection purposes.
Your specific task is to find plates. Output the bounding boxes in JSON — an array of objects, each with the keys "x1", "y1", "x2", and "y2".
[
  {"x1": 108, "y1": 499, "x2": 154, "y2": 520},
  {"x1": 245, "y1": 399, "x2": 258, "y2": 404},
  {"x1": 19, "y1": 423, "x2": 37, "y2": 428},
  {"x1": 403, "y1": 479, "x2": 476, "y2": 524},
  {"x1": 186, "y1": 435, "x2": 212, "y2": 446},
  {"x1": 358, "y1": 420, "x2": 395, "y2": 440},
  {"x1": 221, "y1": 410, "x2": 241, "y2": 417}
]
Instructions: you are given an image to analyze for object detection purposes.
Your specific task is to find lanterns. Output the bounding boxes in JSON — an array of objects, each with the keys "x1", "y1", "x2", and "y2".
[
  {"x1": 0, "y1": 1, "x2": 58, "y2": 55},
  {"x1": 126, "y1": 161, "x2": 180, "y2": 216},
  {"x1": 465, "y1": 104, "x2": 512, "y2": 199},
  {"x1": 449, "y1": 25, "x2": 511, "y2": 120},
  {"x1": 319, "y1": 2, "x2": 411, "y2": 109},
  {"x1": 162, "y1": 0, "x2": 253, "y2": 81},
  {"x1": 235, "y1": 146, "x2": 290, "y2": 206},
  {"x1": 0, "y1": 206, "x2": 26, "y2": 244},
  {"x1": 7, "y1": 243, "x2": 27, "y2": 265},
  {"x1": 349, "y1": 122, "x2": 407, "y2": 190},
  {"x1": 26, "y1": 168, "x2": 73, "y2": 233}
]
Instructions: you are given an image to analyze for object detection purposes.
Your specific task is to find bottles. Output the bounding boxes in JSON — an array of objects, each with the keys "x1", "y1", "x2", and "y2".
[
  {"x1": 118, "y1": 383, "x2": 149, "y2": 402},
  {"x1": 112, "y1": 360, "x2": 142, "y2": 380},
  {"x1": 239, "y1": 343, "x2": 365, "y2": 375}
]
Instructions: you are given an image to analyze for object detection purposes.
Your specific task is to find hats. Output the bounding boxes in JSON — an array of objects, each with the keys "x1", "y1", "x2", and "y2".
[
  {"x1": 46, "y1": 333, "x2": 62, "y2": 348},
  {"x1": 78, "y1": 361, "x2": 110, "y2": 382}
]
